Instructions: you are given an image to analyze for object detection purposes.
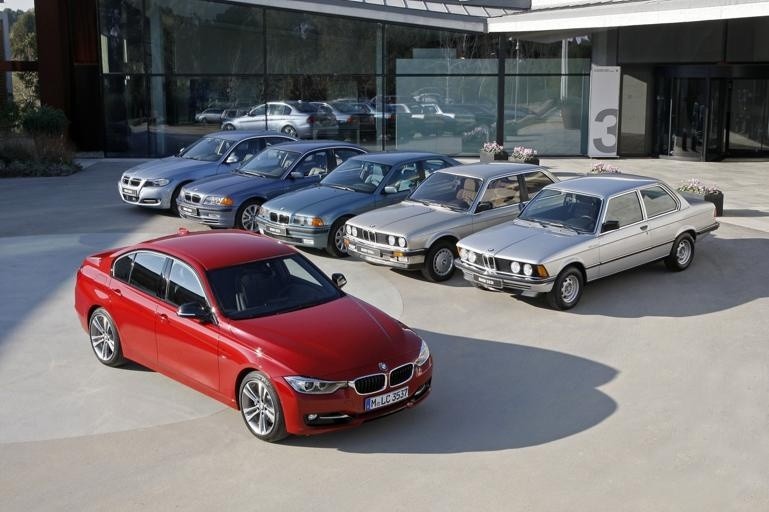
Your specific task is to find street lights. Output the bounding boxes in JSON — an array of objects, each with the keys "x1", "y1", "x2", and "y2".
[
  {"x1": 507, "y1": 36, "x2": 519, "y2": 122},
  {"x1": 442, "y1": 55, "x2": 467, "y2": 105}
]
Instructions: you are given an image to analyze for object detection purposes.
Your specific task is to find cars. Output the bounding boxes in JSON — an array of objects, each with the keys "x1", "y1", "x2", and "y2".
[{"x1": 66, "y1": 224, "x2": 436, "y2": 447}]
